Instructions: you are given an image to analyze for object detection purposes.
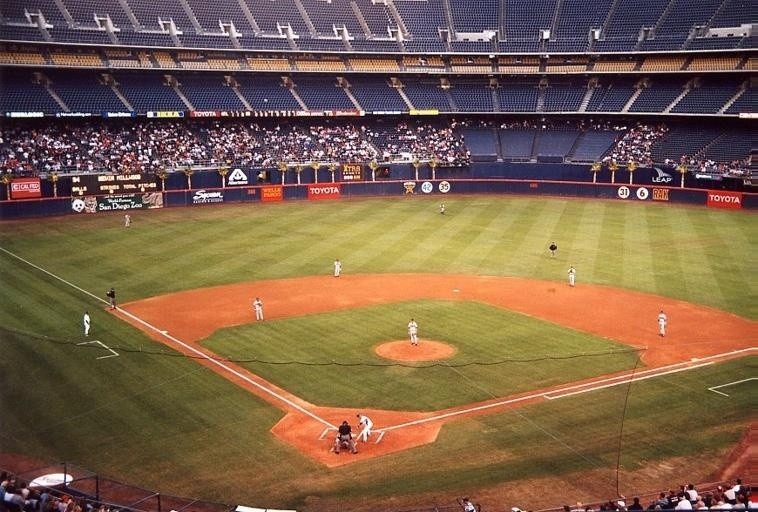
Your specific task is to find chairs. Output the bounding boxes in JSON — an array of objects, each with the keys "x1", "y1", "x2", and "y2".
[{"x1": 0, "y1": 0, "x2": 758, "y2": 182}]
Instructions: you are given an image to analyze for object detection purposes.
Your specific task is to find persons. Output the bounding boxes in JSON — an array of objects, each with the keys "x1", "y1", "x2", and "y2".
[
  {"x1": 333, "y1": 258, "x2": 342, "y2": 277},
  {"x1": 334, "y1": 414, "x2": 374, "y2": 453},
  {"x1": 0, "y1": 472, "x2": 118, "y2": 512},
  {"x1": 408, "y1": 318, "x2": 419, "y2": 346},
  {"x1": 1, "y1": 120, "x2": 752, "y2": 178},
  {"x1": 462, "y1": 477, "x2": 758, "y2": 512},
  {"x1": 549, "y1": 241, "x2": 557, "y2": 257},
  {"x1": 568, "y1": 264, "x2": 577, "y2": 287},
  {"x1": 106, "y1": 287, "x2": 116, "y2": 310},
  {"x1": 82, "y1": 311, "x2": 92, "y2": 335},
  {"x1": 658, "y1": 311, "x2": 668, "y2": 338},
  {"x1": 253, "y1": 297, "x2": 264, "y2": 321}
]
